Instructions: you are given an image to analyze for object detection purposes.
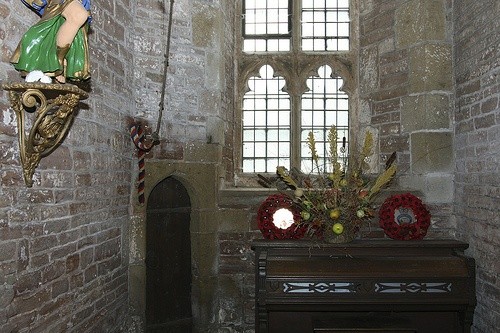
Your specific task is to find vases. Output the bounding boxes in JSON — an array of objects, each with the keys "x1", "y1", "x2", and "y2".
[{"x1": 323, "y1": 229, "x2": 354, "y2": 243}]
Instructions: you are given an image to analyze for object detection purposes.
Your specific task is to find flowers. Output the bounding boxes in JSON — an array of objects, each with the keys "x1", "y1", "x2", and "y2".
[{"x1": 257, "y1": 125, "x2": 396, "y2": 247}]
